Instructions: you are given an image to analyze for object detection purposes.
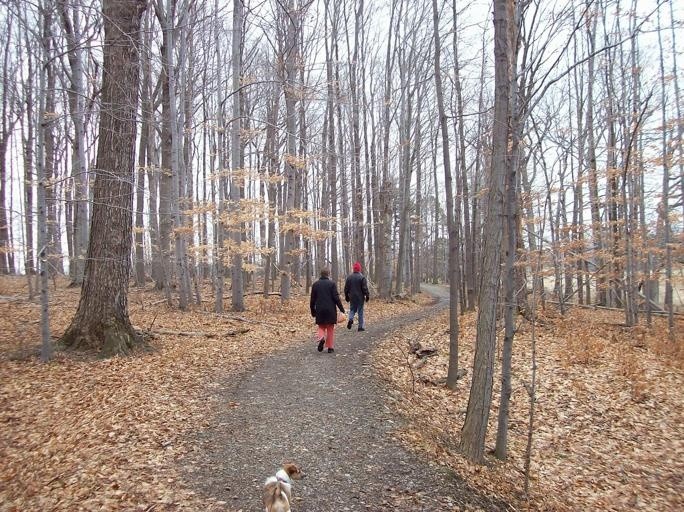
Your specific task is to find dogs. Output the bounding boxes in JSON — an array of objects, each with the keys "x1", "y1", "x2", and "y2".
[{"x1": 262, "y1": 463, "x2": 307, "y2": 512}]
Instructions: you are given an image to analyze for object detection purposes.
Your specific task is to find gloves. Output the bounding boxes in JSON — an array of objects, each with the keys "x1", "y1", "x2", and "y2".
[
  {"x1": 366, "y1": 297, "x2": 369, "y2": 302},
  {"x1": 345, "y1": 296, "x2": 350, "y2": 302}
]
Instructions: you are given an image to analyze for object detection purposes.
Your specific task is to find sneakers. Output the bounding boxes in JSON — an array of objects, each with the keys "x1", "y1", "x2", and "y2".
[
  {"x1": 358, "y1": 328, "x2": 365, "y2": 331},
  {"x1": 347, "y1": 320, "x2": 352, "y2": 329},
  {"x1": 318, "y1": 339, "x2": 325, "y2": 352},
  {"x1": 328, "y1": 348, "x2": 334, "y2": 352}
]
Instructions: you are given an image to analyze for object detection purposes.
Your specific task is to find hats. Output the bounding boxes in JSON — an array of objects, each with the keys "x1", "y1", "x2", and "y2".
[{"x1": 352, "y1": 263, "x2": 361, "y2": 272}]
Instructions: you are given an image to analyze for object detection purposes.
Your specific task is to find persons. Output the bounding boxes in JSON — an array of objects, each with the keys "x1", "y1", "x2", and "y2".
[
  {"x1": 343, "y1": 262, "x2": 369, "y2": 331},
  {"x1": 308, "y1": 266, "x2": 344, "y2": 352}
]
